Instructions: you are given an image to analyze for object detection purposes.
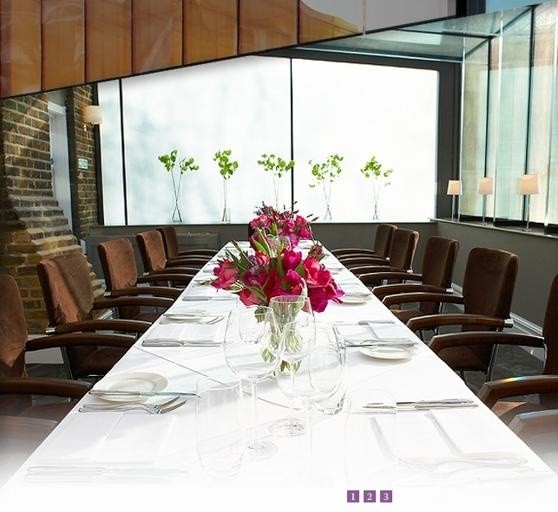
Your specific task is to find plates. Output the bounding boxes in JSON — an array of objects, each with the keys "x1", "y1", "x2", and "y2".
[
  {"x1": 92, "y1": 371, "x2": 168, "y2": 402},
  {"x1": 358, "y1": 342, "x2": 415, "y2": 359},
  {"x1": 339, "y1": 290, "x2": 372, "y2": 303},
  {"x1": 167, "y1": 309, "x2": 211, "y2": 320}
]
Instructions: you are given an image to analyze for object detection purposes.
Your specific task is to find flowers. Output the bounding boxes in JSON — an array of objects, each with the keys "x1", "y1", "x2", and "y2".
[
  {"x1": 200, "y1": 221, "x2": 344, "y2": 371},
  {"x1": 248, "y1": 199, "x2": 318, "y2": 252}
]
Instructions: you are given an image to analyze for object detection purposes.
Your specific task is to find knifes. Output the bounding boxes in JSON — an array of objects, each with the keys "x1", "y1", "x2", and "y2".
[
  {"x1": 89, "y1": 389, "x2": 197, "y2": 395},
  {"x1": 346, "y1": 341, "x2": 420, "y2": 347},
  {"x1": 182, "y1": 296, "x2": 235, "y2": 301},
  {"x1": 361, "y1": 398, "x2": 478, "y2": 411},
  {"x1": 142, "y1": 338, "x2": 222, "y2": 347}
]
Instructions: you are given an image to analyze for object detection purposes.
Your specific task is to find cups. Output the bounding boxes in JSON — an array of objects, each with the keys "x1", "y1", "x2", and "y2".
[
  {"x1": 194, "y1": 372, "x2": 243, "y2": 473},
  {"x1": 261, "y1": 296, "x2": 318, "y2": 437},
  {"x1": 275, "y1": 320, "x2": 348, "y2": 462},
  {"x1": 223, "y1": 305, "x2": 285, "y2": 461}
]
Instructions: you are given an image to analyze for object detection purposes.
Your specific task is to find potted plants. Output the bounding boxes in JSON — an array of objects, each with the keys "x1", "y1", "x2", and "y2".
[
  {"x1": 258, "y1": 153, "x2": 295, "y2": 209},
  {"x1": 156, "y1": 150, "x2": 200, "y2": 222},
  {"x1": 212, "y1": 148, "x2": 238, "y2": 221},
  {"x1": 308, "y1": 154, "x2": 343, "y2": 221},
  {"x1": 360, "y1": 157, "x2": 394, "y2": 220}
]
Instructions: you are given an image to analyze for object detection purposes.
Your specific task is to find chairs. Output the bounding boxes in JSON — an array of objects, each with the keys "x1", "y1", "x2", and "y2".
[
  {"x1": 97, "y1": 239, "x2": 194, "y2": 334},
  {"x1": 157, "y1": 226, "x2": 218, "y2": 286},
  {"x1": 382, "y1": 248, "x2": 519, "y2": 385},
  {"x1": 429, "y1": 274, "x2": 558, "y2": 424},
  {"x1": 137, "y1": 230, "x2": 201, "y2": 313},
  {"x1": 0, "y1": 273, "x2": 135, "y2": 449},
  {"x1": 359, "y1": 236, "x2": 459, "y2": 341},
  {"x1": 331, "y1": 224, "x2": 397, "y2": 288},
  {"x1": 340, "y1": 229, "x2": 419, "y2": 309},
  {"x1": 0, "y1": 413, "x2": 58, "y2": 484},
  {"x1": 37, "y1": 251, "x2": 174, "y2": 403}
]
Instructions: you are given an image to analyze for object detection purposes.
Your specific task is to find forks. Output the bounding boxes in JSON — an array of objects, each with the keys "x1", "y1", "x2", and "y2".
[
  {"x1": 78, "y1": 399, "x2": 188, "y2": 416},
  {"x1": 83, "y1": 395, "x2": 181, "y2": 411}
]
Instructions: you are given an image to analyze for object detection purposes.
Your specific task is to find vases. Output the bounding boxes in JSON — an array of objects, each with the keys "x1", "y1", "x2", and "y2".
[{"x1": 263, "y1": 300, "x2": 307, "y2": 373}]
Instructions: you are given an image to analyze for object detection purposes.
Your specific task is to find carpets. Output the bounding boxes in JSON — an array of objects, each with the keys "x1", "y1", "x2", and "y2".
[{"x1": 26, "y1": 273, "x2": 546, "y2": 406}]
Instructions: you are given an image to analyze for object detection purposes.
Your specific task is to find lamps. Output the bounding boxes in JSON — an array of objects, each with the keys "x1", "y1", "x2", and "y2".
[
  {"x1": 84, "y1": 105, "x2": 103, "y2": 131},
  {"x1": 518, "y1": 173, "x2": 539, "y2": 232},
  {"x1": 477, "y1": 178, "x2": 493, "y2": 224},
  {"x1": 446, "y1": 180, "x2": 462, "y2": 221}
]
